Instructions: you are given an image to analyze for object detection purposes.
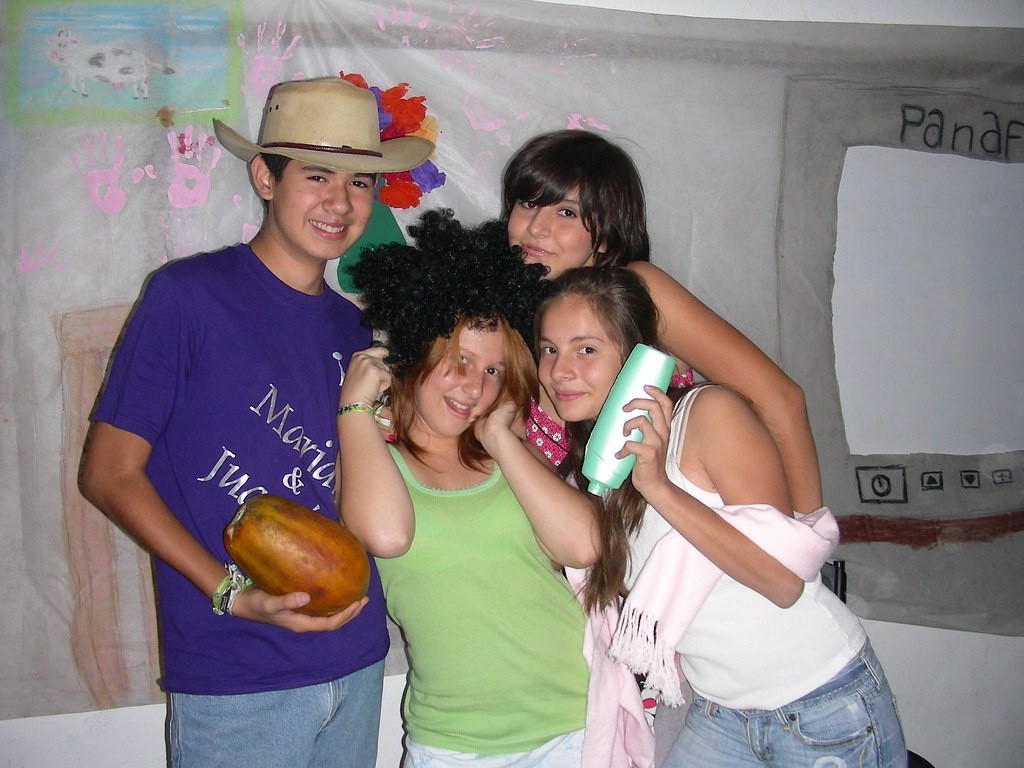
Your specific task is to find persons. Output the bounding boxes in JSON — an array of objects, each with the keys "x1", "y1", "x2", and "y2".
[
  {"x1": 498, "y1": 130, "x2": 908, "y2": 768},
  {"x1": 79, "y1": 75, "x2": 434, "y2": 768},
  {"x1": 335, "y1": 205, "x2": 605, "y2": 768}
]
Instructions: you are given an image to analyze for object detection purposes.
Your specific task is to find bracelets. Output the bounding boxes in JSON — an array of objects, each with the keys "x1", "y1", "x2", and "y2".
[
  {"x1": 334, "y1": 393, "x2": 391, "y2": 429},
  {"x1": 211, "y1": 562, "x2": 254, "y2": 616}
]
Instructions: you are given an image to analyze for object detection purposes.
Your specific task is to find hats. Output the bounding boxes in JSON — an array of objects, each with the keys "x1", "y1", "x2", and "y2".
[{"x1": 210, "y1": 77, "x2": 437, "y2": 173}]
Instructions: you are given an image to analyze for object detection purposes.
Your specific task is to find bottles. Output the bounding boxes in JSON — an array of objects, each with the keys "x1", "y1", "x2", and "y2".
[{"x1": 582, "y1": 345, "x2": 676, "y2": 496}]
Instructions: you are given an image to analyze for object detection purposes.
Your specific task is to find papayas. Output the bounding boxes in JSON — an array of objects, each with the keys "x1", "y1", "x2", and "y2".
[{"x1": 224, "y1": 495, "x2": 369, "y2": 617}]
